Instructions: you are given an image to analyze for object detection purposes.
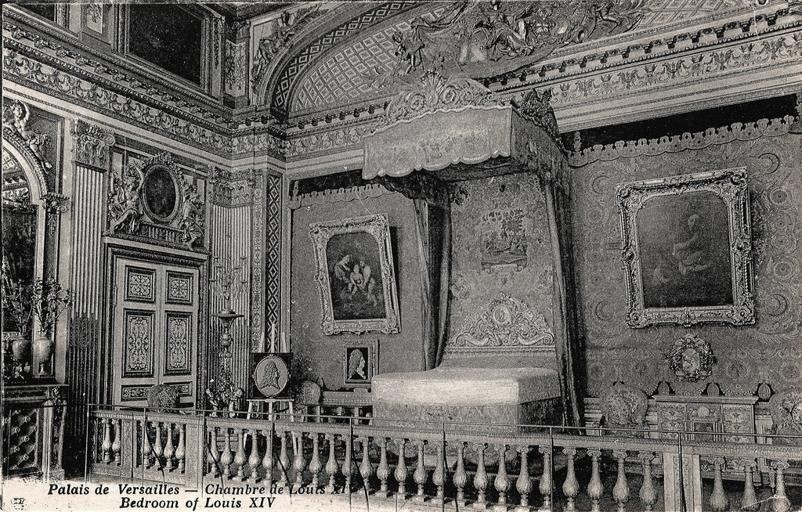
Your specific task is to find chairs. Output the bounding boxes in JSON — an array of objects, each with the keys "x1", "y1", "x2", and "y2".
[{"x1": 145, "y1": 383, "x2": 183, "y2": 442}]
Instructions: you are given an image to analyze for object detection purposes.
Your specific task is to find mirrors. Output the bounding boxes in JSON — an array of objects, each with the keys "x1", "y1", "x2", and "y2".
[{"x1": 0, "y1": 122, "x2": 49, "y2": 385}]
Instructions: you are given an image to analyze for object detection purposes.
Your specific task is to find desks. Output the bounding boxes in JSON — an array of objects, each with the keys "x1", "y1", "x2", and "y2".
[{"x1": 241, "y1": 395, "x2": 300, "y2": 458}]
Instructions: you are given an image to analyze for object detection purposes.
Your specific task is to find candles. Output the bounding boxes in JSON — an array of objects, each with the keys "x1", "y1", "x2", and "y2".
[
  {"x1": 282, "y1": 331, "x2": 287, "y2": 354},
  {"x1": 257, "y1": 332, "x2": 263, "y2": 352},
  {"x1": 270, "y1": 323, "x2": 276, "y2": 352}
]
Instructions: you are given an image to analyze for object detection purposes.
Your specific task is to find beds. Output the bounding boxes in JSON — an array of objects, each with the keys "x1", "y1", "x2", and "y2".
[{"x1": 357, "y1": 68, "x2": 584, "y2": 469}]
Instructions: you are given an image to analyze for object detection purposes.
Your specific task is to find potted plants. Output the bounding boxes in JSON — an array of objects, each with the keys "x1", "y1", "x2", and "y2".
[
  {"x1": 16, "y1": 274, "x2": 74, "y2": 382},
  {"x1": 1, "y1": 264, "x2": 39, "y2": 378}
]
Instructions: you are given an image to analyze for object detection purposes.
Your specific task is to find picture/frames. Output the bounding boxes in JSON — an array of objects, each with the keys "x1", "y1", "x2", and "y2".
[
  {"x1": 340, "y1": 339, "x2": 379, "y2": 389},
  {"x1": 307, "y1": 210, "x2": 402, "y2": 338},
  {"x1": 612, "y1": 165, "x2": 761, "y2": 330}
]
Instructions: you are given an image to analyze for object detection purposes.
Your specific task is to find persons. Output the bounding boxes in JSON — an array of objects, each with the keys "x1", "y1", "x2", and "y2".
[
  {"x1": 259, "y1": 362, "x2": 281, "y2": 390},
  {"x1": 347, "y1": 349, "x2": 367, "y2": 381},
  {"x1": 334, "y1": 252, "x2": 383, "y2": 315},
  {"x1": 172, "y1": 184, "x2": 206, "y2": 251},
  {"x1": 250, "y1": 6, "x2": 320, "y2": 94},
  {"x1": 644, "y1": 209, "x2": 715, "y2": 289},
  {"x1": 101, "y1": 158, "x2": 145, "y2": 238},
  {"x1": 205, "y1": 376, "x2": 244, "y2": 418},
  {"x1": 392, "y1": 1, "x2": 621, "y2": 75},
  {"x1": 3, "y1": 99, "x2": 53, "y2": 175}
]
